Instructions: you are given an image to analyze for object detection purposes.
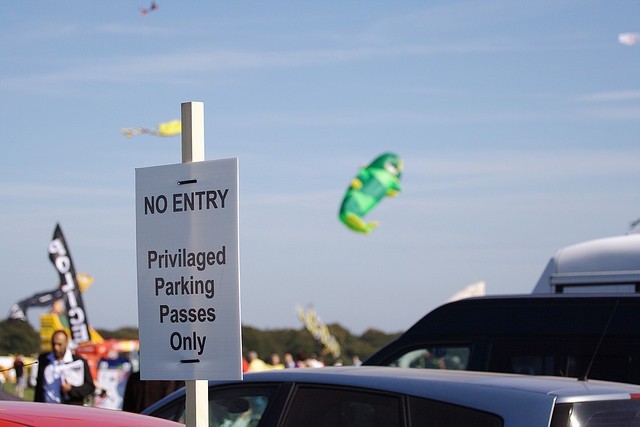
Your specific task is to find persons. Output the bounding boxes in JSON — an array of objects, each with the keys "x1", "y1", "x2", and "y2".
[
  {"x1": 295, "y1": 350, "x2": 308, "y2": 367},
  {"x1": 243, "y1": 349, "x2": 271, "y2": 372},
  {"x1": 266, "y1": 352, "x2": 285, "y2": 369},
  {"x1": 282, "y1": 352, "x2": 298, "y2": 367},
  {"x1": 35, "y1": 330, "x2": 96, "y2": 405}
]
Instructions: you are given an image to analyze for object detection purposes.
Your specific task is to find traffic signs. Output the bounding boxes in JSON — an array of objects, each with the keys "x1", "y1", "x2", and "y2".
[{"x1": 134, "y1": 156, "x2": 244, "y2": 381}]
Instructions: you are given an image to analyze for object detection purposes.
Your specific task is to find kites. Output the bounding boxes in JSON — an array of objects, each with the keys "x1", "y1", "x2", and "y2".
[{"x1": 121, "y1": 116, "x2": 181, "y2": 141}]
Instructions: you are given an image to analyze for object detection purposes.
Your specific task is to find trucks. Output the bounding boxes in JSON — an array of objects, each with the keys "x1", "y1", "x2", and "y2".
[{"x1": 531, "y1": 233, "x2": 640, "y2": 294}]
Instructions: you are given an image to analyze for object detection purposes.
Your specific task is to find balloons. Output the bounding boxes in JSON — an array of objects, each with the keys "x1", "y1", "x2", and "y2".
[{"x1": 337, "y1": 152, "x2": 406, "y2": 236}]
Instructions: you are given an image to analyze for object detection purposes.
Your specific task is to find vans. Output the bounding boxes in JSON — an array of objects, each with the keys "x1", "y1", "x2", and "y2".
[{"x1": 359, "y1": 293, "x2": 639, "y2": 386}]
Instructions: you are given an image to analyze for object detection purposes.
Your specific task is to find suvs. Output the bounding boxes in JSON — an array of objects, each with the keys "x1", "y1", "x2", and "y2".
[{"x1": 140, "y1": 365, "x2": 640, "y2": 426}]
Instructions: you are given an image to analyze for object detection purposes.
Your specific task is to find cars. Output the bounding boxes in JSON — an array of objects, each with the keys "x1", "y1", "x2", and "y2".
[{"x1": 0, "y1": 399, "x2": 185, "y2": 426}]
